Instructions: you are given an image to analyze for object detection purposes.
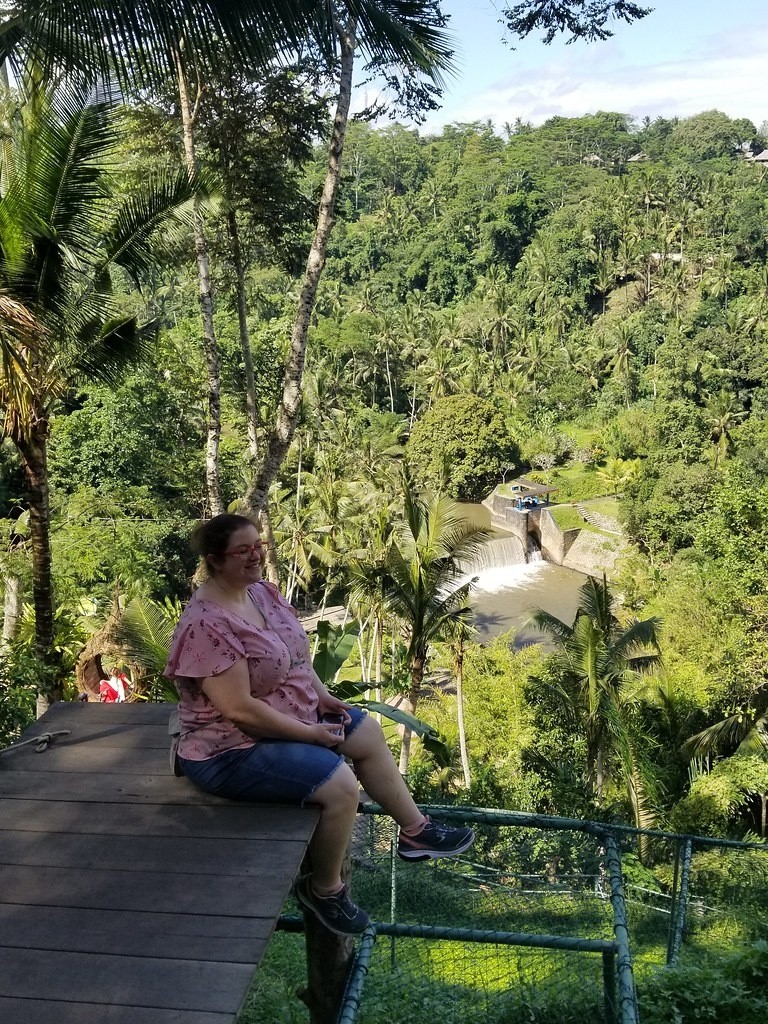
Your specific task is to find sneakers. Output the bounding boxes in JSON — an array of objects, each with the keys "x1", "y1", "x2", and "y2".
[
  {"x1": 398, "y1": 814, "x2": 474, "y2": 862},
  {"x1": 295, "y1": 873, "x2": 369, "y2": 936}
]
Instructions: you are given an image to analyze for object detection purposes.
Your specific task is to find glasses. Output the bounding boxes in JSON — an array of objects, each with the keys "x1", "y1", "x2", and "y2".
[{"x1": 214, "y1": 542, "x2": 269, "y2": 562}]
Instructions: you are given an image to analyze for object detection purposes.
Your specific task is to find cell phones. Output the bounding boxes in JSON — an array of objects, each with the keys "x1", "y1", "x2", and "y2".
[{"x1": 322, "y1": 712, "x2": 344, "y2": 737}]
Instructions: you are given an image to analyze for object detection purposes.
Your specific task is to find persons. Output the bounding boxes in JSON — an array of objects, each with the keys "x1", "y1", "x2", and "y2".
[
  {"x1": 98, "y1": 666, "x2": 132, "y2": 704},
  {"x1": 78, "y1": 692, "x2": 88, "y2": 703},
  {"x1": 162, "y1": 511, "x2": 476, "y2": 938}
]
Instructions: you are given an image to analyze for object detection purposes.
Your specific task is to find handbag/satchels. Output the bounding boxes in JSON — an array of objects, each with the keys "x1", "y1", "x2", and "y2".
[{"x1": 168, "y1": 710, "x2": 181, "y2": 777}]
